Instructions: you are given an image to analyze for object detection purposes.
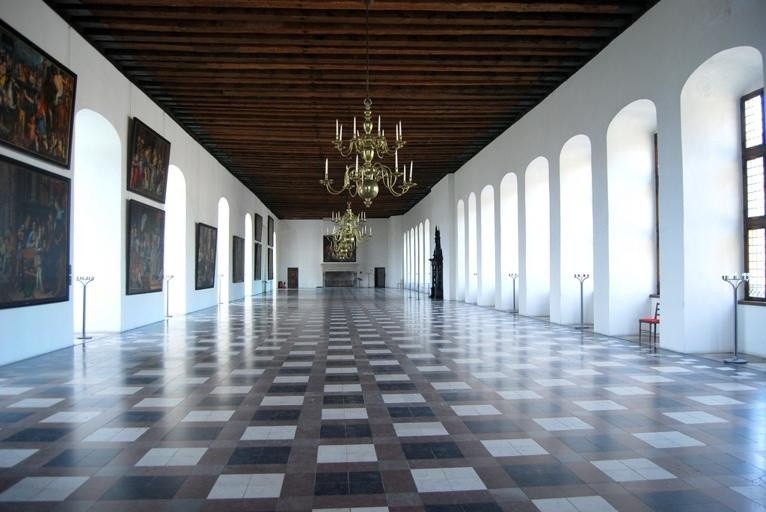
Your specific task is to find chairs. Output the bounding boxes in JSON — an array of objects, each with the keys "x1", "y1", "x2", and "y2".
[{"x1": 638, "y1": 301, "x2": 660, "y2": 343}]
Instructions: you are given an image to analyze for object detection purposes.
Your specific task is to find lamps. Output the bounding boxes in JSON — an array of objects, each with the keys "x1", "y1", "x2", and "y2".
[{"x1": 318, "y1": 1, "x2": 419, "y2": 261}]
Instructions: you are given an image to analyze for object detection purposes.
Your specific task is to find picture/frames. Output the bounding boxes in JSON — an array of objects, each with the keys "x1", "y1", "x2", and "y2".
[
  {"x1": 0, "y1": 154, "x2": 71, "y2": 309},
  {"x1": 0, "y1": 17, "x2": 78, "y2": 170},
  {"x1": 254, "y1": 243, "x2": 262, "y2": 280},
  {"x1": 126, "y1": 116, "x2": 172, "y2": 205},
  {"x1": 255, "y1": 213, "x2": 262, "y2": 242},
  {"x1": 268, "y1": 215, "x2": 274, "y2": 247},
  {"x1": 233, "y1": 236, "x2": 245, "y2": 283},
  {"x1": 126, "y1": 198, "x2": 165, "y2": 295},
  {"x1": 195, "y1": 223, "x2": 217, "y2": 290},
  {"x1": 268, "y1": 248, "x2": 273, "y2": 280}
]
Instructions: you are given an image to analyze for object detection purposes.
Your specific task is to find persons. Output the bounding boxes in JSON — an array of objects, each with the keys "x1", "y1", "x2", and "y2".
[
  {"x1": 0, "y1": 199, "x2": 67, "y2": 296},
  {"x1": 0, "y1": 60, "x2": 72, "y2": 160}
]
